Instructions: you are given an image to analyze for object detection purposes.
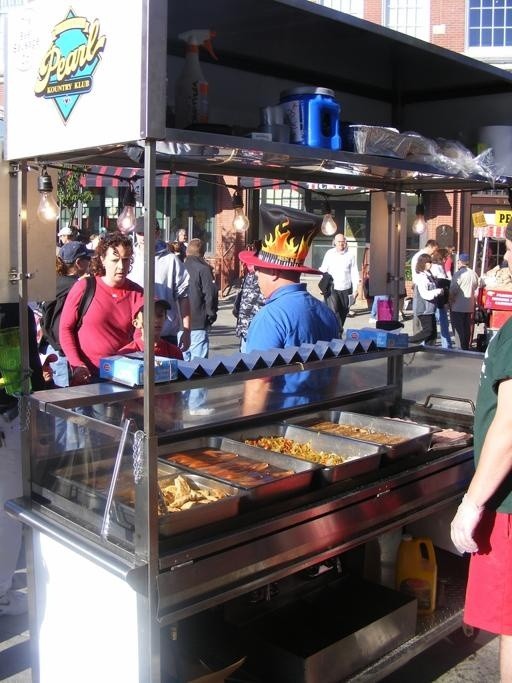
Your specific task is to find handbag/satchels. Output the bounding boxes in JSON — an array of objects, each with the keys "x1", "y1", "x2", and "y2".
[{"x1": 232, "y1": 271, "x2": 250, "y2": 319}]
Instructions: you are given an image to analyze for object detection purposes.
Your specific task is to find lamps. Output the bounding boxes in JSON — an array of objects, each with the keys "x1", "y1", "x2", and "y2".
[
  {"x1": 34, "y1": 160, "x2": 63, "y2": 225},
  {"x1": 315, "y1": 192, "x2": 340, "y2": 237},
  {"x1": 114, "y1": 178, "x2": 142, "y2": 235},
  {"x1": 408, "y1": 187, "x2": 430, "y2": 236},
  {"x1": 228, "y1": 184, "x2": 255, "y2": 234}
]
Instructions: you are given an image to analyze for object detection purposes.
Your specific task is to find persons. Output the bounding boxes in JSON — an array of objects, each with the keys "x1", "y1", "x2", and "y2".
[
  {"x1": 361, "y1": 246, "x2": 374, "y2": 311},
  {"x1": 318, "y1": 232, "x2": 360, "y2": 340},
  {"x1": 166, "y1": 226, "x2": 218, "y2": 362},
  {"x1": 447, "y1": 251, "x2": 480, "y2": 351},
  {"x1": 57, "y1": 230, "x2": 144, "y2": 449},
  {"x1": 116, "y1": 292, "x2": 184, "y2": 442},
  {"x1": 448, "y1": 214, "x2": 512, "y2": 683},
  {"x1": 125, "y1": 215, "x2": 192, "y2": 352},
  {"x1": 22, "y1": 223, "x2": 109, "y2": 510},
  {"x1": 406, "y1": 239, "x2": 459, "y2": 349},
  {"x1": 240, "y1": 265, "x2": 343, "y2": 416},
  {"x1": 231, "y1": 243, "x2": 266, "y2": 353}
]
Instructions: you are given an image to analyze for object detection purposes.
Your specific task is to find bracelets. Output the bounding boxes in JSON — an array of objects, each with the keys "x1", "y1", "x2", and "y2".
[{"x1": 459, "y1": 495, "x2": 488, "y2": 513}]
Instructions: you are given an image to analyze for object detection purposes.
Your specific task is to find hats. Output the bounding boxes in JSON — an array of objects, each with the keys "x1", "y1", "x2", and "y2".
[
  {"x1": 56, "y1": 225, "x2": 75, "y2": 237},
  {"x1": 458, "y1": 253, "x2": 471, "y2": 262},
  {"x1": 129, "y1": 294, "x2": 173, "y2": 321},
  {"x1": 505, "y1": 216, "x2": 512, "y2": 242},
  {"x1": 236, "y1": 202, "x2": 326, "y2": 276},
  {"x1": 37, "y1": 347, "x2": 60, "y2": 369},
  {"x1": 56, "y1": 240, "x2": 99, "y2": 265},
  {"x1": 132, "y1": 215, "x2": 160, "y2": 234}
]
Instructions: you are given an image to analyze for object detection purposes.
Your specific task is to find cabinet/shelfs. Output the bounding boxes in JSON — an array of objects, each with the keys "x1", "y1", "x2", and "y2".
[
  {"x1": 2, "y1": 0, "x2": 512, "y2": 191},
  {"x1": 0, "y1": 329, "x2": 489, "y2": 682}
]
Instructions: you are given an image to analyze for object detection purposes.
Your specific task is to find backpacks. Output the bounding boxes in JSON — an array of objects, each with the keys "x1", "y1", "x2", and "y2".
[
  {"x1": 37, "y1": 274, "x2": 98, "y2": 358},
  {"x1": 428, "y1": 269, "x2": 451, "y2": 309}
]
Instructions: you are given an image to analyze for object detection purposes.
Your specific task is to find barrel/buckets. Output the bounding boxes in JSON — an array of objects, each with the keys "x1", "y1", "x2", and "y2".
[{"x1": 395, "y1": 536, "x2": 439, "y2": 615}]
